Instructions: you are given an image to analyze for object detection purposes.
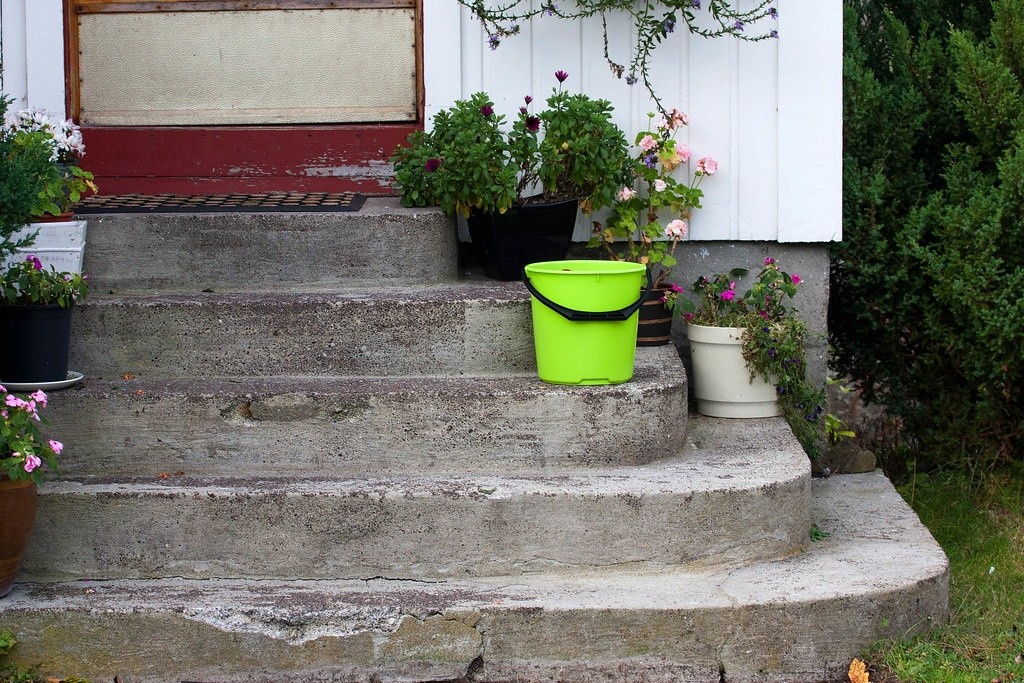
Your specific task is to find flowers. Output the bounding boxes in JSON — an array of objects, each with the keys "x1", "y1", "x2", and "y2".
[
  {"x1": 662, "y1": 253, "x2": 856, "y2": 477},
  {"x1": 391, "y1": 70, "x2": 638, "y2": 217},
  {"x1": 0, "y1": 384, "x2": 65, "y2": 482},
  {"x1": 586, "y1": 110, "x2": 718, "y2": 290},
  {"x1": 0, "y1": 254, "x2": 90, "y2": 307},
  {"x1": 5, "y1": 109, "x2": 98, "y2": 217}
]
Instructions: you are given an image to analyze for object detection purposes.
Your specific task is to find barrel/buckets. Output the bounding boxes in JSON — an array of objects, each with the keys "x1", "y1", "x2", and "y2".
[{"x1": 522, "y1": 260, "x2": 647, "y2": 386}]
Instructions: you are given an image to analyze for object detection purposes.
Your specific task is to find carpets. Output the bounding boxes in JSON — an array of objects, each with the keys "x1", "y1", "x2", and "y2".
[{"x1": 59, "y1": 192, "x2": 366, "y2": 214}]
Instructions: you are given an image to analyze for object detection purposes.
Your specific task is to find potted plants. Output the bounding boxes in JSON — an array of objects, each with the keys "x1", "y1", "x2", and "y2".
[{"x1": 0, "y1": 93, "x2": 88, "y2": 301}]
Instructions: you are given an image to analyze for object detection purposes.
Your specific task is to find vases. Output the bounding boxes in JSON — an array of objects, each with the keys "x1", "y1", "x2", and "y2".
[
  {"x1": 31, "y1": 212, "x2": 73, "y2": 222},
  {"x1": 467, "y1": 195, "x2": 579, "y2": 282},
  {"x1": 637, "y1": 283, "x2": 676, "y2": 347},
  {"x1": 0, "y1": 297, "x2": 85, "y2": 392},
  {"x1": 687, "y1": 321, "x2": 785, "y2": 419},
  {"x1": 0, "y1": 470, "x2": 39, "y2": 599}
]
